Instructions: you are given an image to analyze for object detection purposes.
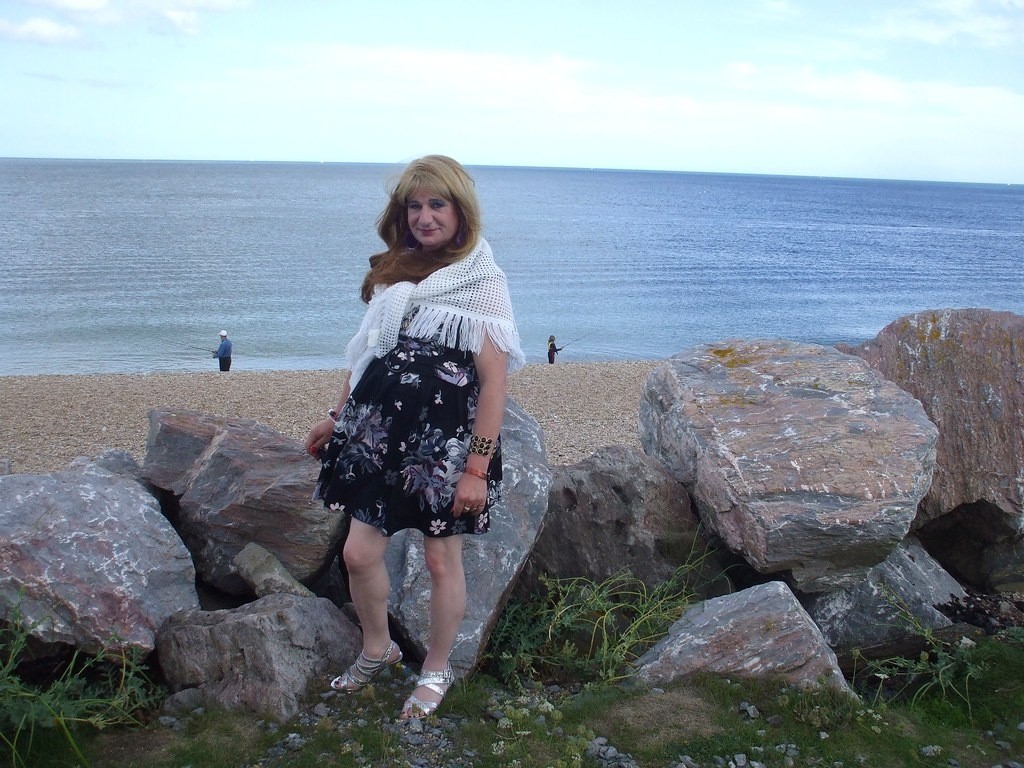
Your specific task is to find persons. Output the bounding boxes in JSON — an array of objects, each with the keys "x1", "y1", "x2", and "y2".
[
  {"x1": 211, "y1": 330, "x2": 232, "y2": 371},
  {"x1": 547, "y1": 335, "x2": 562, "y2": 363},
  {"x1": 305, "y1": 155, "x2": 527, "y2": 720}
]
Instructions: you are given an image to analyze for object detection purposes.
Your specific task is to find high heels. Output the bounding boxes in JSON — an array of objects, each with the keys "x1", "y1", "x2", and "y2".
[
  {"x1": 331, "y1": 640, "x2": 403, "y2": 692},
  {"x1": 399, "y1": 661, "x2": 455, "y2": 722}
]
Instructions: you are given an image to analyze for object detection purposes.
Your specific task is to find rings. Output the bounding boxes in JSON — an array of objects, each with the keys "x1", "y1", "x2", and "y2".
[
  {"x1": 472, "y1": 507, "x2": 476, "y2": 510},
  {"x1": 464, "y1": 506, "x2": 470, "y2": 511}
]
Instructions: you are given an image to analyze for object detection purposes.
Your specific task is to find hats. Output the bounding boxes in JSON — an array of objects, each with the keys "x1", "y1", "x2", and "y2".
[{"x1": 219, "y1": 329, "x2": 227, "y2": 336}]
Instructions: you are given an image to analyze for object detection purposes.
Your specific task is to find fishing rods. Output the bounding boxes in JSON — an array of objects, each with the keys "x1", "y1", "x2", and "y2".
[
  {"x1": 152, "y1": 336, "x2": 215, "y2": 353},
  {"x1": 556, "y1": 327, "x2": 605, "y2": 352}
]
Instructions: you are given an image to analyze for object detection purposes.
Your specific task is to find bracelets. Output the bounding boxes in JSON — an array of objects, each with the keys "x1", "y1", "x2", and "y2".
[
  {"x1": 463, "y1": 467, "x2": 488, "y2": 481},
  {"x1": 328, "y1": 409, "x2": 338, "y2": 424},
  {"x1": 469, "y1": 435, "x2": 497, "y2": 459}
]
